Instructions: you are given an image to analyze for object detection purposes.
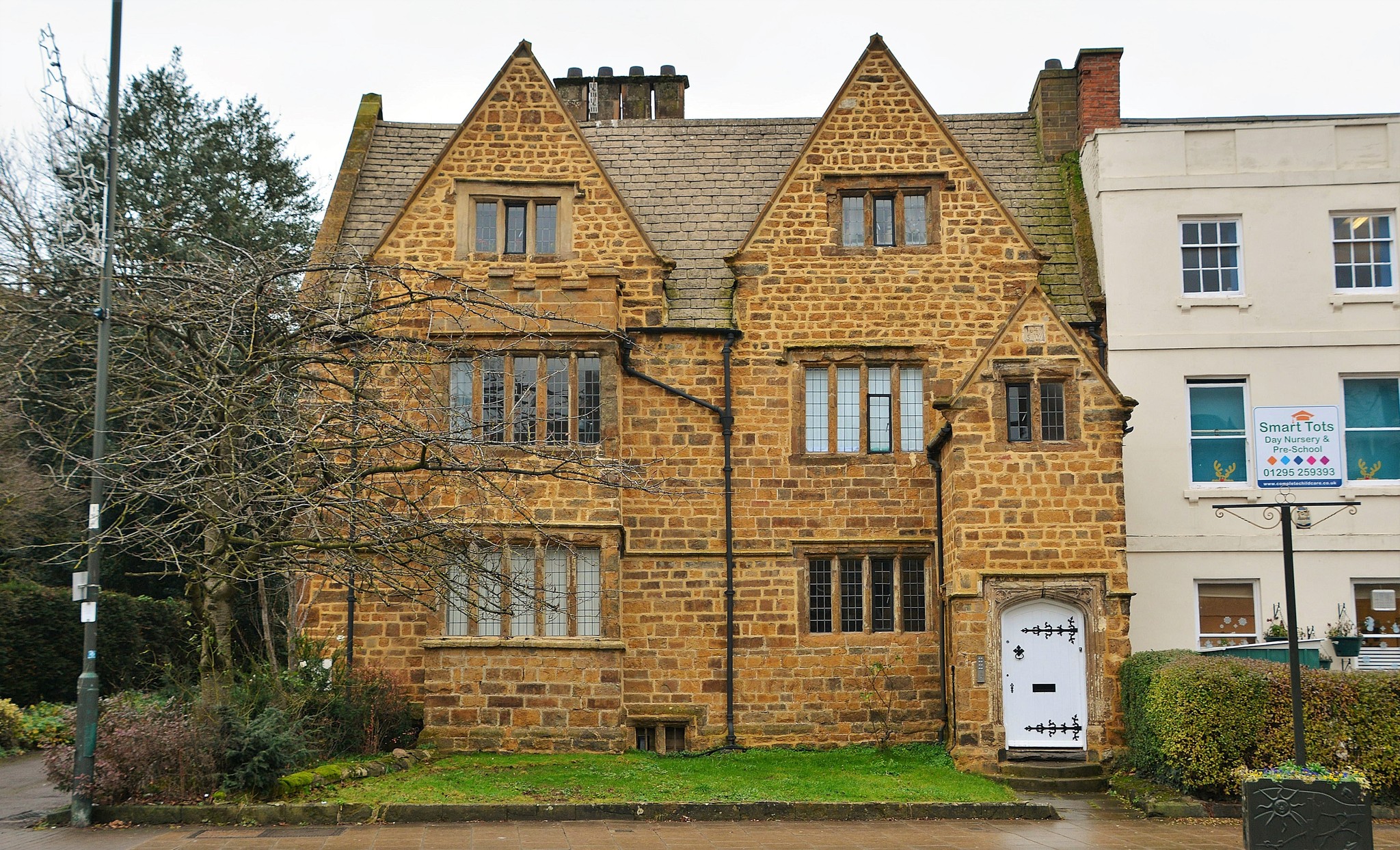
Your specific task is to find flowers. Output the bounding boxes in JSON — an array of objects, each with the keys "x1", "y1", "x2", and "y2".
[{"x1": 1263, "y1": 619, "x2": 1287, "y2": 636}]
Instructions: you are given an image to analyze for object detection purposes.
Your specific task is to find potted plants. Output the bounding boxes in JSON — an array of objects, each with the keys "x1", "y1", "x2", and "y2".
[{"x1": 1325, "y1": 621, "x2": 1366, "y2": 658}]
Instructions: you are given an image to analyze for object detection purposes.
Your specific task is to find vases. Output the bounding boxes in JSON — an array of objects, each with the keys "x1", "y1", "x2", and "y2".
[{"x1": 1264, "y1": 637, "x2": 1290, "y2": 642}]
